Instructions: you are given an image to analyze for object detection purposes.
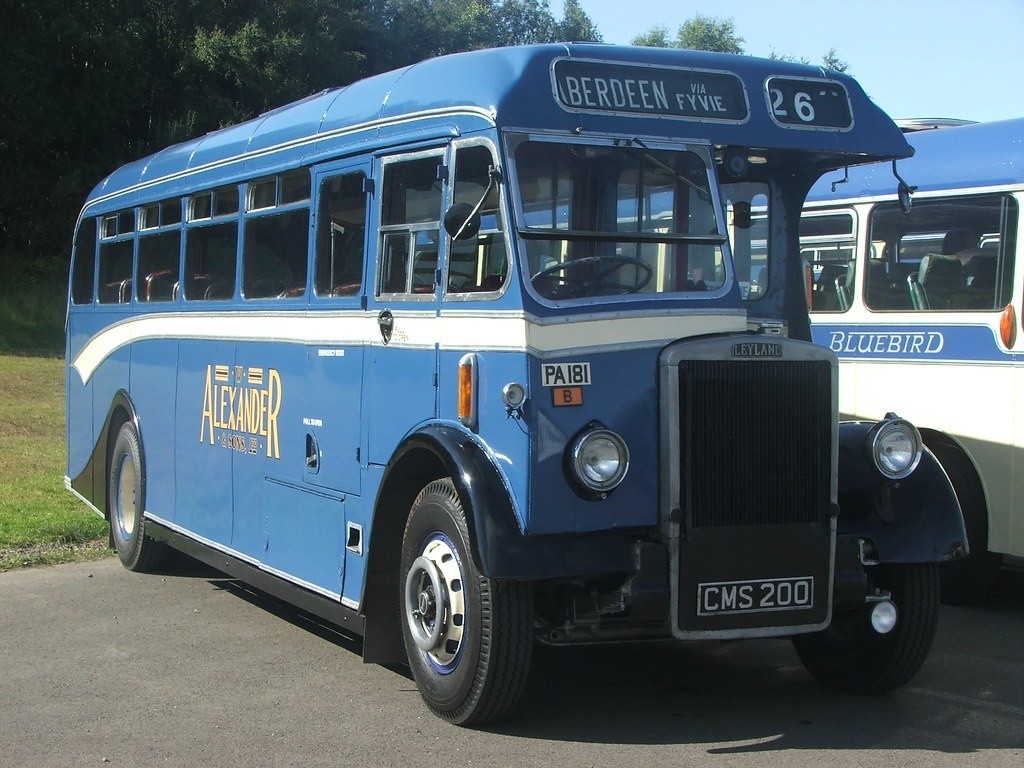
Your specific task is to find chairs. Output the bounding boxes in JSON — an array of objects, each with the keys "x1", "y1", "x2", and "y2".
[
  {"x1": 807, "y1": 255, "x2": 997, "y2": 309},
  {"x1": 101, "y1": 262, "x2": 501, "y2": 304}
]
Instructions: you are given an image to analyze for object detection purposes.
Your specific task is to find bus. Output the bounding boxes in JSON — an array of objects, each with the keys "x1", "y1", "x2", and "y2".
[
  {"x1": 63, "y1": 44, "x2": 972, "y2": 727},
  {"x1": 404, "y1": 115, "x2": 1023, "y2": 603}
]
